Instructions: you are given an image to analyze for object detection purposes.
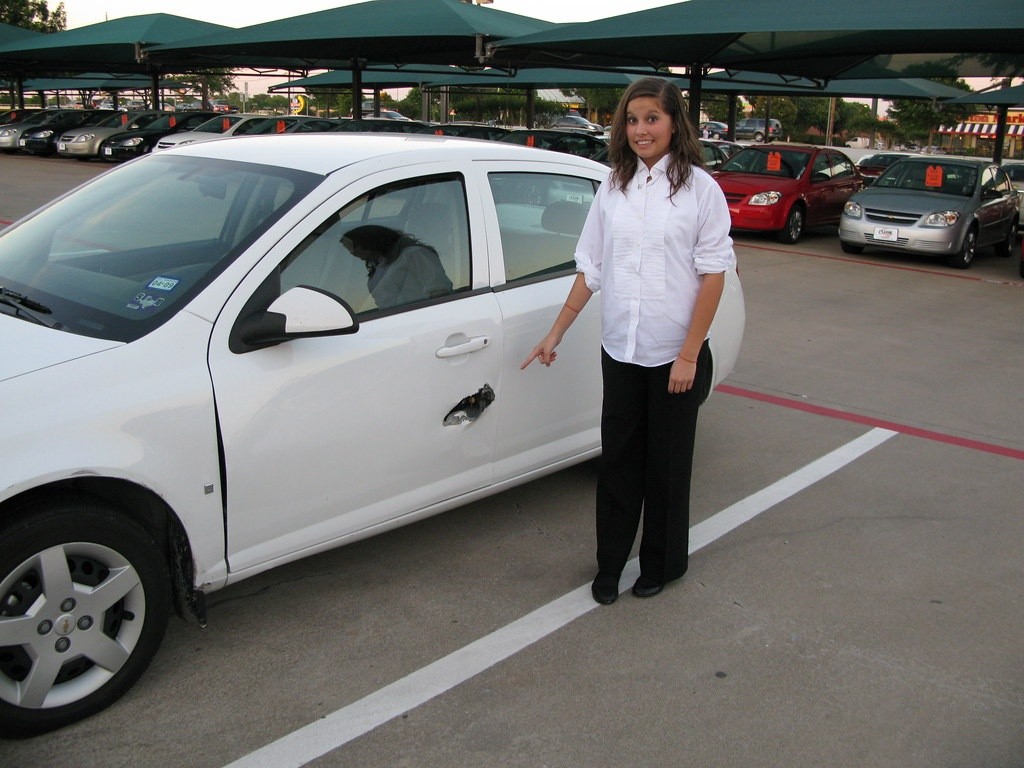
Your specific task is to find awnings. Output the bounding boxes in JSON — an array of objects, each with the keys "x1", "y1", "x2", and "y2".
[{"x1": 0, "y1": 0, "x2": 1024, "y2": 164}]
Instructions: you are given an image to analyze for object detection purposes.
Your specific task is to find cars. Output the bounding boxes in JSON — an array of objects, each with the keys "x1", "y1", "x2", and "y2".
[
  {"x1": 699, "y1": 121, "x2": 728, "y2": 139},
  {"x1": 151, "y1": 114, "x2": 276, "y2": 163},
  {"x1": 48, "y1": 100, "x2": 239, "y2": 113},
  {"x1": 0, "y1": 110, "x2": 60, "y2": 152},
  {"x1": 350, "y1": 101, "x2": 398, "y2": 118},
  {"x1": 368, "y1": 111, "x2": 413, "y2": 121},
  {"x1": 101, "y1": 112, "x2": 227, "y2": 162},
  {"x1": 418, "y1": 125, "x2": 511, "y2": 141},
  {"x1": 549, "y1": 140, "x2": 731, "y2": 212},
  {"x1": 332, "y1": 119, "x2": 426, "y2": 133},
  {"x1": 56, "y1": 110, "x2": 179, "y2": 161},
  {"x1": 735, "y1": 118, "x2": 783, "y2": 142},
  {"x1": 19, "y1": 110, "x2": 113, "y2": 155},
  {"x1": 551, "y1": 116, "x2": 604, "y2": 136},
  {"x1": 488, "y1": 130, "x2": 608, "y2": 204},
  {"x1": 0, "y1": 110, "x2": 44, "y2": 126},
  {"x1": 243, "y1": 116, "x2": 344, "y2": 135},
  {"x1": 709, "y1": 143, "x2": 865, "y2": 244},
  {"x1": 0, "y1": 131, "x2": 746, "y2": 738},
  {"x1": 838, "y1": 154, "x2": 1020, "y2": 268}
]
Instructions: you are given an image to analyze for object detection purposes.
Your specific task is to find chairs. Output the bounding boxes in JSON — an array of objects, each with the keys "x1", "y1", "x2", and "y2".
[
  {"x1": 500, "y1": 200, "x2": 588, "y2": 280},
  {"x1": 349, "y1": 192, "x2": 461, "y2": 318}
]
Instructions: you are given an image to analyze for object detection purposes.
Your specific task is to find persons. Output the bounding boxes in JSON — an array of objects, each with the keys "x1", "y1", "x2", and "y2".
[
  {"x1": 340, "y1": 225, "x2": 453, "y2": 310},
  {"x1": 521, "y1": 76, "x2": 734, "y2": 605}
]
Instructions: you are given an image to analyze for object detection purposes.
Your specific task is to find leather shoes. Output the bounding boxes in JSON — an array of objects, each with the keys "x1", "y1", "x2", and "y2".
[
  {"x1": 633, "y1": 575, "x2": 666, "y2": 597},
  {"x1": 592, "y1": 571, "x2": 620, "y2": 605}
]
